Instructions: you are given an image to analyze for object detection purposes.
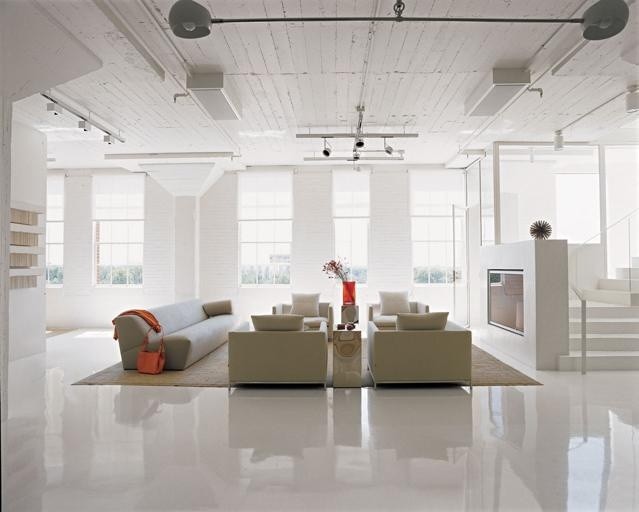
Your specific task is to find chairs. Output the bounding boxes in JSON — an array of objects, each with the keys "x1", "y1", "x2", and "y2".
[
  {"x1": 367, "y1": 390, "x2": 473, "y2": 449},
  {"x1": 228, "y1": 320, "x2": 327, "y2": 391},
  {"x1": 368, "y1": 300, "x2": 428, "y2": 329},
  {"x1": 367, "y1": 318, "x2": 472, "y2": 391},
  {"x1": 227, "y1": 390, "x2": 327, "y2": 449},
  {"x1": 272, "y1": 301, "x2": 333, "y2": 342}
]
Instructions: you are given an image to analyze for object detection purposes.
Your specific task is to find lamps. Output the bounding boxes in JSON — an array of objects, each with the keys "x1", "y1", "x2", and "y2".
[
  {"x1": 79, "y1": 120, "x2": 92, "y2": 132},
  {"x1": 383, "y1": 135, "x2": 393, "y2": 155},
  {"x1": 354, "y1": 125, "x2": 365, "y2": 147},
  {"x1": 169, "y1": 1, "x2": 212, "y2": 39},
  {"x1": 353, "y1": 146, "x2": 360, "y2": 160},
  {"x1": 554, "y1": 131, "x2": 564, "y2": 152},
  {"x1": 625, "y1": 85, "x2": 638, "y2": 114},
  {"x1": 104, "y1": 135, "x2": 115, "y2": 146},
  {"x1": 322, "y1": 136, "x2": 332, "y2": 157},
  {"x1": 47, "y1": 103, "x2": 62, "y2": 118},
  {"x1": 581, "y1": 1, "x2": 629, "y2": 41}
]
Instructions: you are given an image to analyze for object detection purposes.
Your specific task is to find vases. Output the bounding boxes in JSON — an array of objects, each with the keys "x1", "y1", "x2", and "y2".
[{"x1": 343, "y1": 282, "x2": 355, "y2": 305}]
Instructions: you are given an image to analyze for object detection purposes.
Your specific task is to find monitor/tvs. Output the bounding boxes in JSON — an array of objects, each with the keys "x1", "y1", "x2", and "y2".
[{"x1": 488, "y1": 270, "x2": 523, "y2": 336}]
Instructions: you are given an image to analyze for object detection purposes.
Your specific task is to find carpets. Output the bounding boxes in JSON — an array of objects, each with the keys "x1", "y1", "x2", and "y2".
[{"x1": 72, "y1": 328, "x2": 541, "y2": 388}]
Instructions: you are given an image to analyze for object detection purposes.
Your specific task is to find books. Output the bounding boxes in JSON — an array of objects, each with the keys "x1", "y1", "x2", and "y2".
[{"x1": 11, "y1": 205, "x2": 38, "y2": 289}]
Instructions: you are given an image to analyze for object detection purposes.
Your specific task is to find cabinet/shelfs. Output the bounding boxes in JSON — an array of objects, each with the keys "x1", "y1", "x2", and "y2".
[{"x1": 9, "y1": 208, "x2": 46, "y2": 289}]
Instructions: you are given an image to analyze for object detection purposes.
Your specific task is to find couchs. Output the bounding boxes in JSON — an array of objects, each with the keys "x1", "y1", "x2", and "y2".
[
  {"x1": 115, "y1": 298, "x2": 248, "y2": 371},
  {"x1": 114, "y1": 385, "x2": 203, "y2": 425}
]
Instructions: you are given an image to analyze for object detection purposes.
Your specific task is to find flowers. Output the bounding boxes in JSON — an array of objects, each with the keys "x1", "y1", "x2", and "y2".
[{"x1": 322, "y1": 259, "x2": 356, "y2": 303}]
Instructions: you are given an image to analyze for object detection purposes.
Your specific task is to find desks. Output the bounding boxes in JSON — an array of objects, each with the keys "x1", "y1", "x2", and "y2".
[
  {"x1": 333, "y1": 387, "x2": 362, "y2": 447},
  {"x1": 342, "y1": 305, "x2": 360, "y2": 325},
  {"x1": 333, "y1": 323, "x2": 362, "y2": 389}
]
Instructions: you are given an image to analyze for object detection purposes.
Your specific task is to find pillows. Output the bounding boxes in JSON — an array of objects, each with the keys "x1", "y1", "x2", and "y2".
[
  {"x1": 396, "y1": 312, "x2": 448, "y2": 330},
  {"x1": 397, "y1": 448, "x2": 447, "y2": 462},
  {"x1": 378, "y1": 290, "x2": 408, "y2": 314},
  {"x1": 251, "y1": 315, "x2": 304, "y2": 331},
  {"x1": 251, "y1": 449, "x2": 302, "y2": 462},
  {"x1": 291, "y1": 292, "x2": 320, "y2": 316}
]
